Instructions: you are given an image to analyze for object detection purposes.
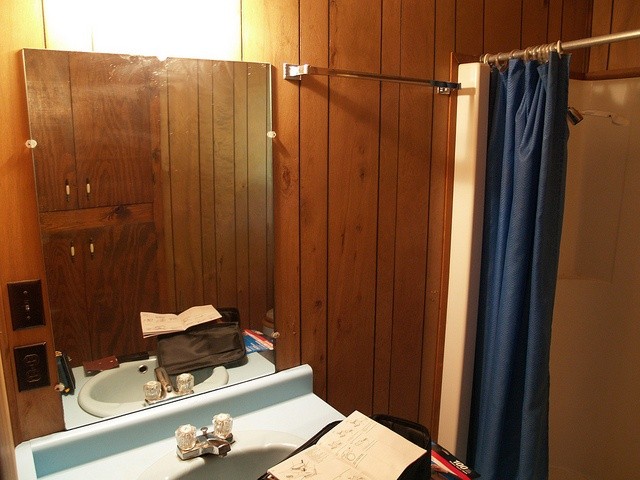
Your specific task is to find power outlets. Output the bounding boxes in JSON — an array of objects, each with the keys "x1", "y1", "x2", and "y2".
[
  {"x1": 13, "y1": 340, "x2": 51, "y2": 392},
  {"x1": 6, "y1": 278, "x2": 47, "y2": 332}
]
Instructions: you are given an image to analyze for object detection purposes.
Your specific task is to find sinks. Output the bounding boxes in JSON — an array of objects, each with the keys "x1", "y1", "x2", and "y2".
[
  {"x1": 89, "y1": 356, "x2": 215, "y2": 406},
  {"x1": 163, "y1": 443, "x2": 299, "y2": 480}
]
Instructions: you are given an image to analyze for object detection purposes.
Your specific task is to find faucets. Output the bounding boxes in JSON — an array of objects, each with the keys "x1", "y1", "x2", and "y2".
[
  {"x1": 176, "y1": 412, "x2": 235, "y2": 460},
  {"x1": 141, "y1": 367, "x2": 193, "y2": 403}
]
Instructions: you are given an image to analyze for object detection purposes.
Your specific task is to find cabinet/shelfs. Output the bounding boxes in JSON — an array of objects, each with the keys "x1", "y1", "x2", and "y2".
[
  {"x1": 42, "y1": 219, "x2": 158, "y2": 365},
  {"x1": 25, "y1": 49, "x2": 154, "y2": 212}
]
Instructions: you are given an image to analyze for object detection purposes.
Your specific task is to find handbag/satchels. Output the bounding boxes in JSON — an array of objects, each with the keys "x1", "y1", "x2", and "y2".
[
  {"x1": 256, "y1": 412, "x2": 432, "y2": 480},
  {"x1": 158, "y1": 306, "x2": 247, "y2": 374}
]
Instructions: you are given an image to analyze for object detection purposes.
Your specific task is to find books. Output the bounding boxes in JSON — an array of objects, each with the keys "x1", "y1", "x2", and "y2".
[
  {"x1": 265, "y1": 409, "x2": 429, "y2": 480},
  {"x1": 139, "y1": 303, "x2": 223, "y2": 340}
]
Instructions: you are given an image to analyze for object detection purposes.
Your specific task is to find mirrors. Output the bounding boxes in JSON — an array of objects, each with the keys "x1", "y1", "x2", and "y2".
[{"x1": 21, "y1": 48, "x2": 278, "y2": 431}]
